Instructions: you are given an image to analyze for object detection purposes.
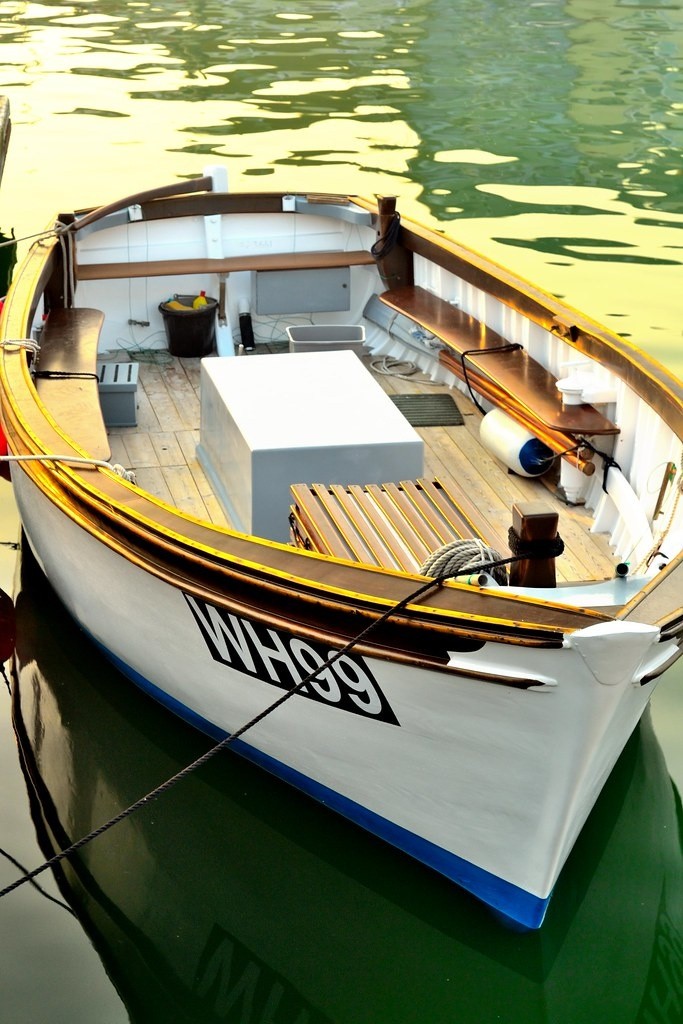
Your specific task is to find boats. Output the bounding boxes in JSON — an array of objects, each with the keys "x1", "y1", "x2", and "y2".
[
  {"x1": 9, "y1": 519, "x2": 683, "y2": 1024},
  {"x1": 0, "y1": 177, "x2": 683, "y2": 937}
]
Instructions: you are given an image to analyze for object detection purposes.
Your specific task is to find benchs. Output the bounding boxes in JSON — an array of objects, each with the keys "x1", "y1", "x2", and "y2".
[
  {"x1": 379, "y1": 284, "x2": 621, "y2": 436},
  {"x1": 286, "y1": 473, "x2": 513, "y2": 575},
  {"x1": 34, "y1": 307, "x2": 112, "y2": 462}
]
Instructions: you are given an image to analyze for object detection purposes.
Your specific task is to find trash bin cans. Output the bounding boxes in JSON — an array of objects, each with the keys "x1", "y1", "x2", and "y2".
[{"x1": 287, "y1": 323, "x2": 367, "y2": 358}]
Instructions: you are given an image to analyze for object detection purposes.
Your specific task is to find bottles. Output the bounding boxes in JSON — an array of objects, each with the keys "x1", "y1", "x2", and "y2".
[{"x1": 193, "y1": 291, "x2": 207, "y2": 309}]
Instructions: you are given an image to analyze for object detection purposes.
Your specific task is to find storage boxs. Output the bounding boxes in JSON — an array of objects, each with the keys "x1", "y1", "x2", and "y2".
[{"x1": 286, "y1": 325, "x2": 366, "y2": 364}]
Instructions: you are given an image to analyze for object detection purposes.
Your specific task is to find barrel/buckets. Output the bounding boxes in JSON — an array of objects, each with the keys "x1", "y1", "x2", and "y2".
[{"x1": 158, "y1": 294, "x2": 218, "y2": 357}]
